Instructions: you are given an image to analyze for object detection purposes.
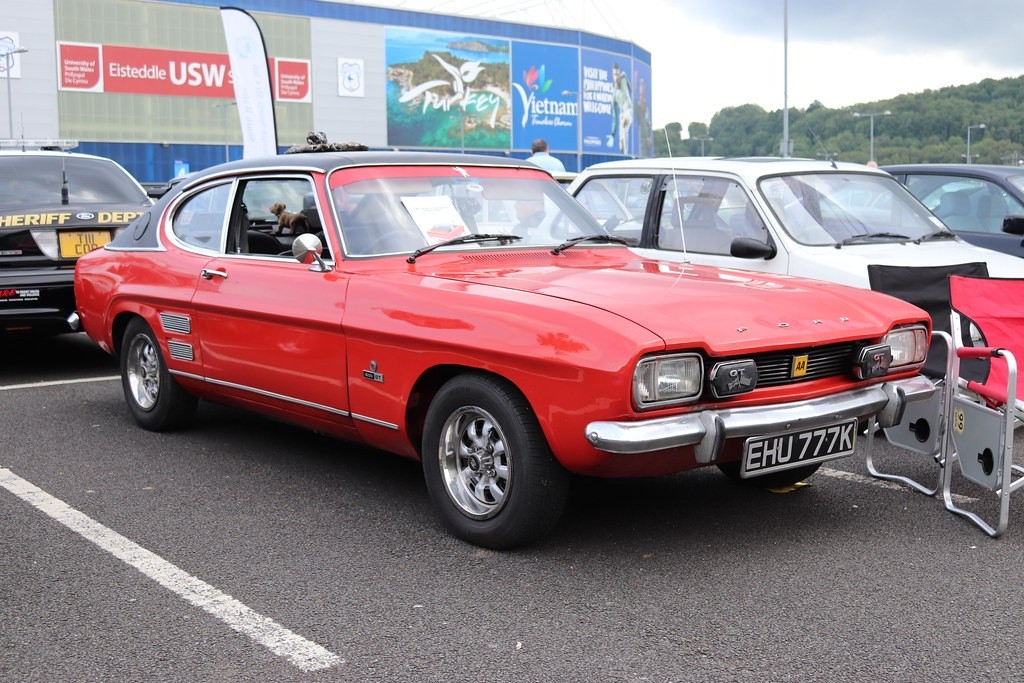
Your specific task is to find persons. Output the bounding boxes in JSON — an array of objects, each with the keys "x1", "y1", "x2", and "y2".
[
  {"x1": 634, "y1": 72, "x2": 654, "y2": 157},
  {"x1": 606, "y1": 63, "x2": 634, "y2": 154},
  {"x1": 524, "y1": 139, "x2": 566, "y2": 174}
]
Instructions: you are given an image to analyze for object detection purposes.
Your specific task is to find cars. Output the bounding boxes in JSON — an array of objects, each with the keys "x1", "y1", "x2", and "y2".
[
  {"x1": 438, "y1": 155, "x2": 1024, "y2": 378},
  {"x1": 0, "y1": 148, "x2": 158, "y2": 340},
  {"x1": 72, "y1": 148, "x2": 937, "y2": 552}
]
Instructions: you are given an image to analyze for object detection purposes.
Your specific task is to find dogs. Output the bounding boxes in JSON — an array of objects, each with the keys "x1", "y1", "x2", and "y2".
[{"x1": 269, "y1": 202, "x2": 310, "y2": 236}]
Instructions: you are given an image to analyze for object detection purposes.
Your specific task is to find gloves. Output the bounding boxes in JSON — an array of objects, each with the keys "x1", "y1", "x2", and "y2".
[{"x1": 606, "y1": 135, "x2": 614, "y2": 147}]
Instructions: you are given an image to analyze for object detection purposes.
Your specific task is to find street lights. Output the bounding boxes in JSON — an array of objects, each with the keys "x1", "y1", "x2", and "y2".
[
  {"x1": 965, "y1": 123, "x2": 985, "y2": 164},
  {"x1": 692, "y1": 135, "x2": 715, "y2": 155},
  {"x1": 851, "y1": 109, "x2": 893, "y2": 162}
]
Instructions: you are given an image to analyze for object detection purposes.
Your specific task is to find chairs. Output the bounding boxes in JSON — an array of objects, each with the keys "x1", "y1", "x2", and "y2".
[
  {"x1": 938, "y1": 192, "x2": 1010, "y2": 234},
  {"x1": 314, "y1": 227, "x2": 372, "y2": 261},
  {"x1": 865, "y1": 262, "x2": 1024, "y2": 537},
  {"x1": 248, "y1": 232, "x2": 285, "y2": 255},
  {"x1": 667, "y1": 196, "x2": 785, "y2": 259}
]
